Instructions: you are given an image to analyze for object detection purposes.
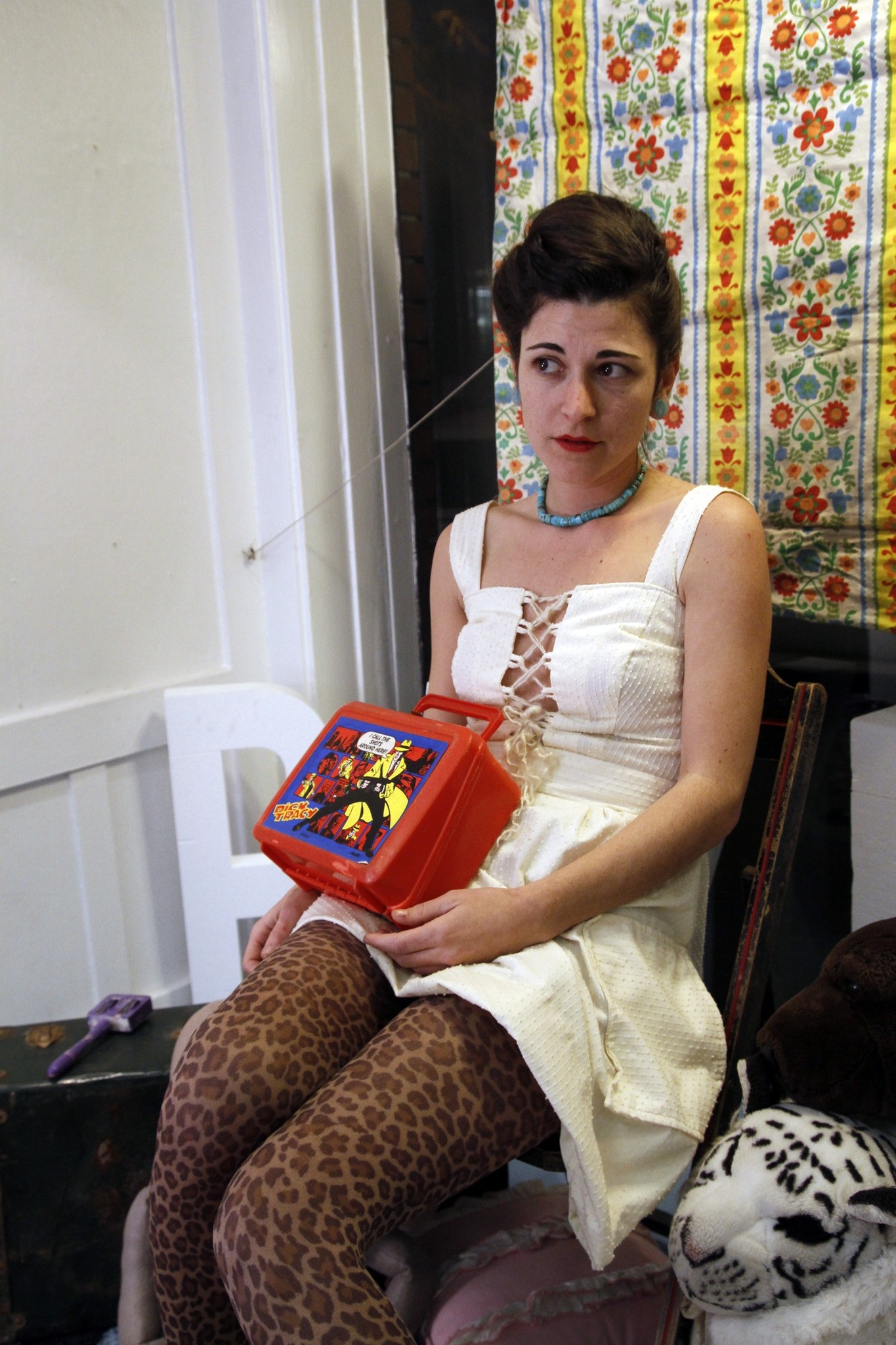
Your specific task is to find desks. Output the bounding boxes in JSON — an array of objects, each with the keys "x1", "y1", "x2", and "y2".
[{"x1": 0, "y1": 1006, "x2": 204, "y2": 1345}]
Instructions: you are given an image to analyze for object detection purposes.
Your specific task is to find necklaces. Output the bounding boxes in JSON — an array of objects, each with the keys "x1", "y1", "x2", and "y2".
[{"x1": 537, "y1": 460, "x2": 647, "y2": 528}]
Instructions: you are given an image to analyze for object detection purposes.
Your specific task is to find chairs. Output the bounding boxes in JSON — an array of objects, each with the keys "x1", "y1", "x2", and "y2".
[{"x1": 458, "y1": 681, "x2": 827, "y2": 1345}]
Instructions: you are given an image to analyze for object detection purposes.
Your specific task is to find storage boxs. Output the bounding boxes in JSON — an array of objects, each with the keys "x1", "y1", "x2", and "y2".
[{"x1": 253, "y1": 692, "x2": 524, "y2": 927}]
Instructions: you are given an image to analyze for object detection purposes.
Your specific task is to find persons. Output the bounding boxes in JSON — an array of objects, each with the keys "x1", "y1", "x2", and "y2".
[{"x1": 144, "y1": 193, "x2": 772, "y2": 1345}]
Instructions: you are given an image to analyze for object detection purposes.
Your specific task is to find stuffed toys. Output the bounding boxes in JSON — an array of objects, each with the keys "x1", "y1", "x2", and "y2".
[
  {"x1": 668, "y1": 1057, "x2": 896, "y2": 1345},
  {"x1": 754, "y1": 917, "x2": 896, "y2": 1123}
]
